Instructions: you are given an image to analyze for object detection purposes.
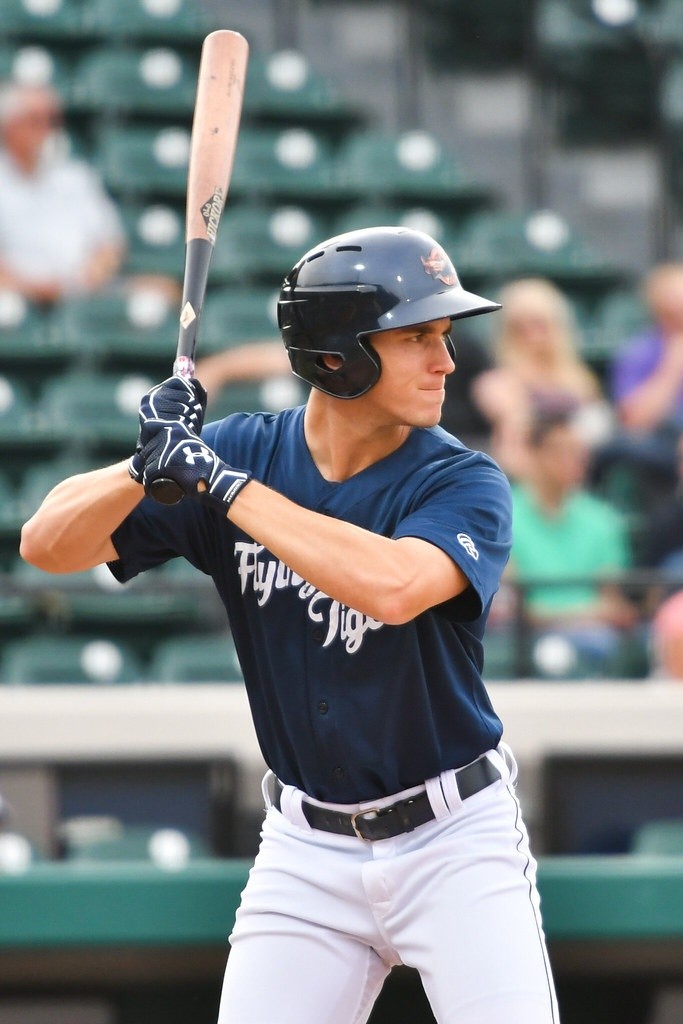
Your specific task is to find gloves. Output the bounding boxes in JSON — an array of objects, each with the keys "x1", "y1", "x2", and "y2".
[
  {"x1": 129, "y1": 416, "x2": 253, "y2": 519},
  {"x1": 128, "y1": 377, "x2": 208, "y2": 480}
]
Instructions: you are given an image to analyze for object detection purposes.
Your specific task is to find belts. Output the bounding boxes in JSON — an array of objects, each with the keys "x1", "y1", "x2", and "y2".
[{"x1": 274, "y1": 756, "x2": 501, "y2": 843}]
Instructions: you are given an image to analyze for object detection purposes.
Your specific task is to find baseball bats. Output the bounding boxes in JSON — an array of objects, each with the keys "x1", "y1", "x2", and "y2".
[{"x1": 146, "y1": 27, "x2": 251, "y2": 508}]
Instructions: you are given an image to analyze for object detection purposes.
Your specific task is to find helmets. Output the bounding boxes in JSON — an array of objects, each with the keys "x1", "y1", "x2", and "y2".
[{"x1": 278, "y1": 226, "x2": 502, "y2": 399}]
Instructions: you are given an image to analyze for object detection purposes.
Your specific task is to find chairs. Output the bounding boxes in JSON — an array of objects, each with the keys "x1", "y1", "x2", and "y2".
[{"x1": 0, "y1": 0, "x2": 683, "y2": 858}]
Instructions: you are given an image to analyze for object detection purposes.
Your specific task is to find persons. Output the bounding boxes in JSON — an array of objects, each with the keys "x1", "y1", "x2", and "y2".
[
  {"x1": 20, "y1": 229, "x2": 561, "y2": 1024},
  {"x1": 476, "y1": 262, "x2": 683, "y2": 681},
  {"x1": 0, "y1": 75, "x2": 180, "y2": 326}
]
